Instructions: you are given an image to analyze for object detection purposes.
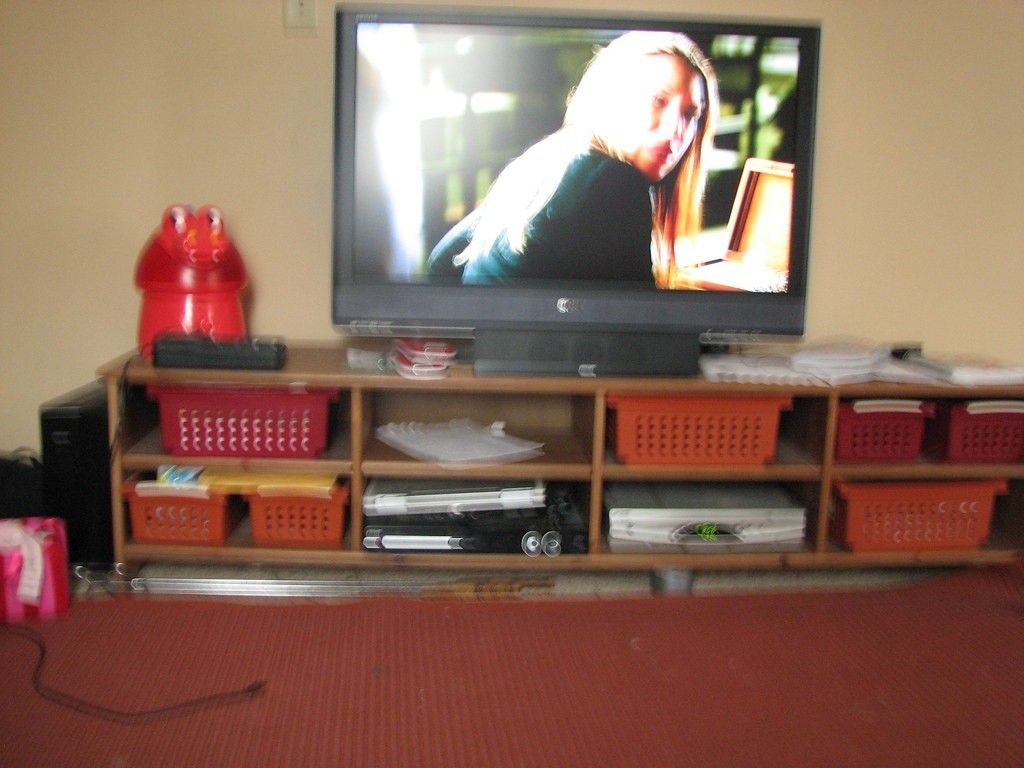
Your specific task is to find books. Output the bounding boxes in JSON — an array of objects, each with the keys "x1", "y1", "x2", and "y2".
[{"x1": 703, "y1": 340, "x2": 1024, "y2": 414}]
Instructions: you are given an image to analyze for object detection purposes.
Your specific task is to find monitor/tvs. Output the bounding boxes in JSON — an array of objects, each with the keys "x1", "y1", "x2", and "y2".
[{"x1": 328, "y1": 7, "x2": 822, "y2": 377}]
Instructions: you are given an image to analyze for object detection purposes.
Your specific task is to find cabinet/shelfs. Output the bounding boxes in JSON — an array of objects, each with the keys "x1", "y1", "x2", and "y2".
[{"x1": 96, "y1": 338, "x2": 1024, "y2": 593}]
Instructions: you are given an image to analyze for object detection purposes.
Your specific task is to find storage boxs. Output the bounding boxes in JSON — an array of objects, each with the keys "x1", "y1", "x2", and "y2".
[
  {"x1": 802, "y1": 479, "x2": 1009, "y2": 555},
  {"x1": 796, "y1": 399, "x2": 935, "y2": 465},
  {"x1": 605, "y1": 393, "x2": 793, "y2": 465},
  {"x1": 143, "y1": 377, "x2": 339, "y2": 459},
  {"x1": 924, "y1": 399, "x2": 1024, "y2": 462},
  {"x1": 241, "y1": 474, "x2": 350, "y2": 547},
  {"x1": 121, "y1": 472, "x2": 239, "y2": 544}
]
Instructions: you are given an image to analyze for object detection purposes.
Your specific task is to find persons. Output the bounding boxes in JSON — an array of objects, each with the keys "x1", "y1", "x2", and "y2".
[{"x1": 426, "y1": 31, "x2": 718, "y2": 289}]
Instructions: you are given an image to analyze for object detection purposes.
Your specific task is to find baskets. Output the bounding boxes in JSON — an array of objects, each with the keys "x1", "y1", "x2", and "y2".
[
  {"x1": 832, "y1": 482, "x2": 1008, "y2": 551},
  {"x1": 243, "y1": 480, "x2": 349, "y2": 550},
  {"x1": 606, "y1": 396, "x2": 791, "y2": 465},
  {"x1": 123, "y1": 473, "x2": 228, "y2": 545},
  {"x1": 927, "y1": 400, "x2": 1024, "y2": 463},
  {"x1": 808, "y1": 399, "x2": 936, "y2": 463},
  {"x1": 149, "y1": 384, "x2": 340, "y2": 458}
]
